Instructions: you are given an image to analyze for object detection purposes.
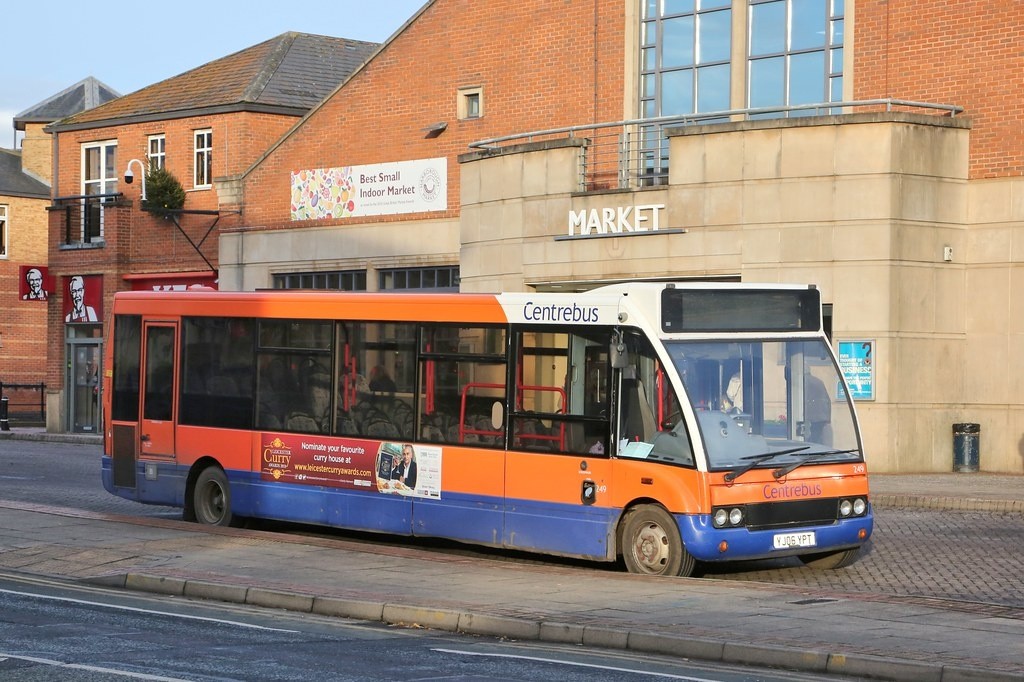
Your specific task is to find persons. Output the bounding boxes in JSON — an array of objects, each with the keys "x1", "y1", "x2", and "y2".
[
  {"x1": 803, "y1": 362, "x2": 831, "y2": 444},
  {"x1": 369, "y1": 365, "x2": 397, "y2": 393},
  {"x1": 392, "y1": 445, "x2": 417, "y2": 490}
]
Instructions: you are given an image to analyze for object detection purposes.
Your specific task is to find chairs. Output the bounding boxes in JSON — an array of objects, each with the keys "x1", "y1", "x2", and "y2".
[
  {"x1": 616, "y1": 377, "x2": 658, "y2": 452},
  {"x1": 256, "y1": 352, "x2": 572, "y2": 454}
]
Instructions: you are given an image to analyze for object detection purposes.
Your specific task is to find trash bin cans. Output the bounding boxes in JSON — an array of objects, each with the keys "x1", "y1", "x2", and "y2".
[{"x1": 952, "y1": 423, "x2": 980, "y2": 474}]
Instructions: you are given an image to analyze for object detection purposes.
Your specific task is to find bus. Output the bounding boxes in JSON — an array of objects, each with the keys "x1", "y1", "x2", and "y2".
[{"x1": 98, "y1": 277, "x2": 874, "y2": 580}]
